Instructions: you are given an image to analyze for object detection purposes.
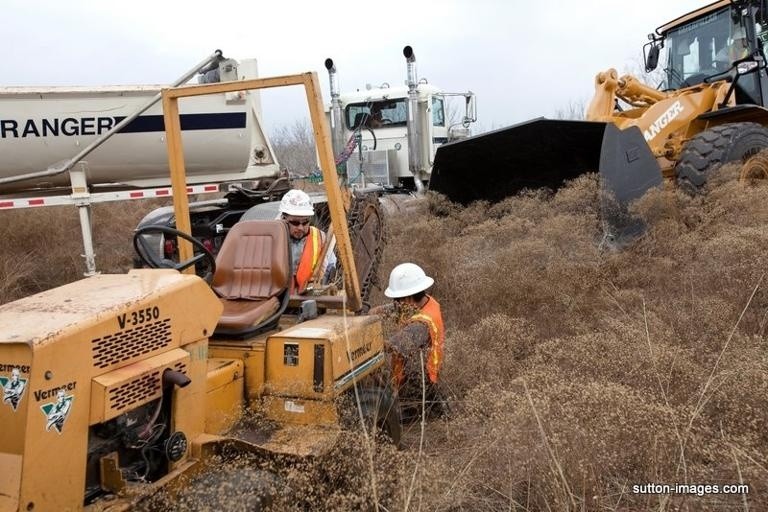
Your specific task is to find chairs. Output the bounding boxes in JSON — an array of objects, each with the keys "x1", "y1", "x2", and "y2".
[
  {"x1": 352, "y1": 114, "x2": 371, "y2": 128},
  {"x1": 203, "y1": 220, "x2": 291, "y2": 340}
]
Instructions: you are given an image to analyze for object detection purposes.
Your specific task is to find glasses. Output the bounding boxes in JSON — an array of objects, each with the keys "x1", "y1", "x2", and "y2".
[{"x1": 281, "y1": 215, "x2": 309, "y2": 226}]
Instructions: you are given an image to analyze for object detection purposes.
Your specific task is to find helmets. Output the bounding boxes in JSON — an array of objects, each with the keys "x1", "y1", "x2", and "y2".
[
  {"x1": 279, "y1": 190, "x2": 315, "y2": 217},
  {"x1": 384, "y1": 263, "x2": 435, "y2": 299}
]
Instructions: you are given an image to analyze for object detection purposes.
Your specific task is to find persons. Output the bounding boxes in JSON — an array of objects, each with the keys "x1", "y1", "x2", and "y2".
[
  {"x1": 275, "y1": 187, "x2": 339, "y2": 294},
  {"x1": 363, "y1": 107, "x2": 383, "y2": 130},
  {"x1": 362, "y1": 261, "x2": 460, "y2": 432},
  {"x1": 714, "y1": 27, "x2": 754, "y2": 71}
]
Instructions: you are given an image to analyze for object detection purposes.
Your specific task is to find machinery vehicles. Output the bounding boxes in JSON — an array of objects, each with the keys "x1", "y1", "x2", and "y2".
[
  {"x1": 315, "y1": 45, "x2": 476, "y2": 196},
  {"x1": 427, "y1": 1, "x2": 766, "y2": 255},
  {"x1": 2, "y1": 52, "x2": 402, "y2": 512}
]
